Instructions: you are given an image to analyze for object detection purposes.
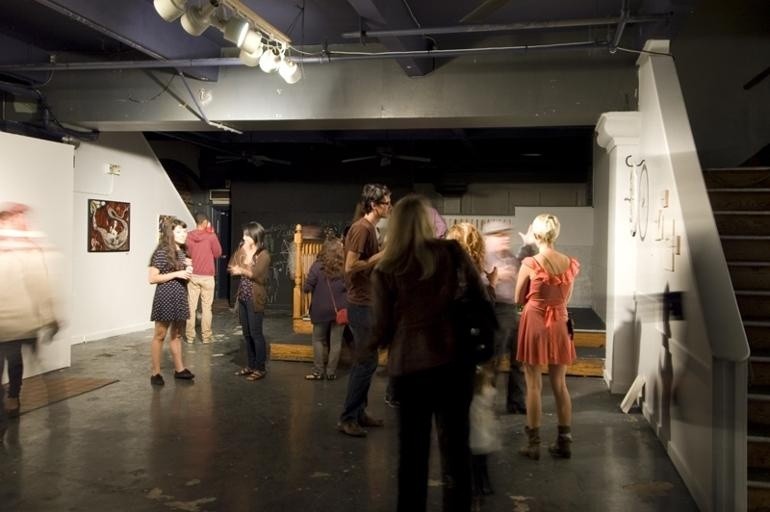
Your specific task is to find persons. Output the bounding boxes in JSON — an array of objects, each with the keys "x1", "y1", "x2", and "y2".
[
  {"x1": 1, "y1": 203, "x2": 68, "y2": 416},
  {"x1": 185, "y1": 212, "x2": 221, "y2": 343},
  {"x1": 149, "y1": 220, "x2": 196, "y2": 385},
  {"x1": 229, "y1": 222, "x2": 272, "y2": 380},
  {"x1": 363, "y1": 196, "x2": 505, "y2": 510},
  {"x1": 0, "y1": 410, "x2": 70, "y2": 512},
  {"x1": 150, "y1": 379, "x2": 213, "y2": 501},
  {"x1": 303, "y1": 185, "x2": 398, "y2": 438},
  {"x1": 427, "y1": 209, "x2": 581, "y2": 461}
]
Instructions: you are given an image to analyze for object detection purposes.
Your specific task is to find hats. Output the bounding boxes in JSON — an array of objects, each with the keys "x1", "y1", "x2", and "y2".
[{"x1": 482, "y1": 221, "x2": 513, "y2": 235}]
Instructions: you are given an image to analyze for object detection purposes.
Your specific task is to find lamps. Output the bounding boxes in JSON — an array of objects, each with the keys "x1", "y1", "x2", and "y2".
[
  {"x1": 106, "y1": 163, "x2": 123, "y2": 179},
  {"x1": 145, "y1": 0, "x2": 303, "y2": 89}
]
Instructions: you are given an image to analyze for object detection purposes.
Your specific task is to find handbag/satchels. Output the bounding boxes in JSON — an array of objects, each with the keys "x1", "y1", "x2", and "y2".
[
  {"x1": 567, "y1": 318, "x2": 575, "y2": 334},
  {"x1": 336, "y1": 309, "x2": 349, "y2": 325}
]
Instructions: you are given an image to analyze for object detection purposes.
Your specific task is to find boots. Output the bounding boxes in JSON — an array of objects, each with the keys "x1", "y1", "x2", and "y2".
[
  {"x1": 520, "y1": 424, "x2": 540, "y2": 459},
  {"x1": 549, "y1": 424, "x2": 573, "y2": 459}
]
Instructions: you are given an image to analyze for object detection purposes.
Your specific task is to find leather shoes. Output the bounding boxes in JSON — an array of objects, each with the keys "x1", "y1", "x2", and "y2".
[
  {"x1": 151, "y1": 374, "x2": 164, "y2": 385},
  {"x1": 356, "y1": 407, "x2": 385, "y2": 426},
  {"x1": 175, "y1": 369, "x2": 195, "y2": 379},
  {"x1": 336, "y1": 419, "x2": 368, "y2": 437}
]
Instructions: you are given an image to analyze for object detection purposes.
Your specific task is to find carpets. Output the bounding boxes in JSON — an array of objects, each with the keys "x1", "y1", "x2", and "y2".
[{"x1": 0, "y1": 372, "x2": 121, "y2": 428}]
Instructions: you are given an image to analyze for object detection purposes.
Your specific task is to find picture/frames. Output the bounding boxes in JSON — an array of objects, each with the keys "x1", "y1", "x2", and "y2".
[{"x1": 87, "y1": 197, "x2": 132, "y2": 254}]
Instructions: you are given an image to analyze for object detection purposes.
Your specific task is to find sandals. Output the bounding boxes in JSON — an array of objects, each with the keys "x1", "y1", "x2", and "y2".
[
  {"x1": 247, "y1": 370, "x2": 267, "y2": 381},
  {"x1": 305, "y1": 373, "x2": 324, "y2": 380},
  {"x1": 327, "y1": 375, "x2": 336, "y2": 380},
  {"x1": 233, "y1": 367, "x2": 253, "y2": 376}
]
